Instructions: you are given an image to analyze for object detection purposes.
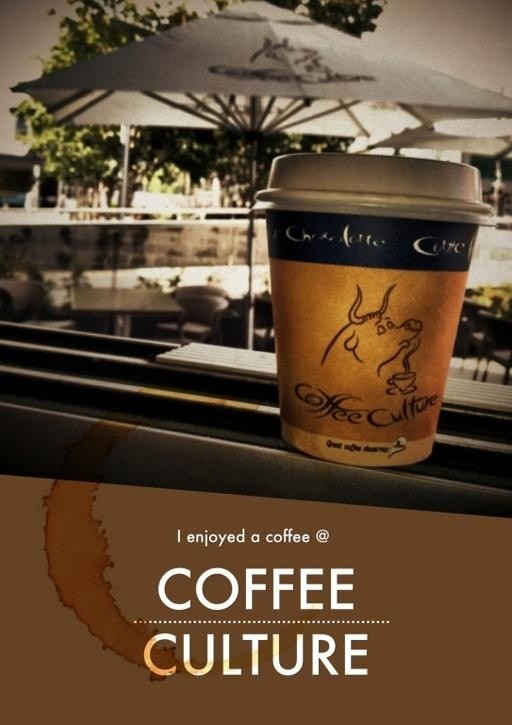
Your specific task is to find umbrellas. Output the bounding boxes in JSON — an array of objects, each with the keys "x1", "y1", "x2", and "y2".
[{"x1": 11, "y1": 3, "x2": 511, "y2": 356}]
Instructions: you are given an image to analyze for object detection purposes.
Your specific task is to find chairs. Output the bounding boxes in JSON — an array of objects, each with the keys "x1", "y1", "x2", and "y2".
[
  {"x1": 0, "y1": 276, "x2": 77, "y2": 330},
  {"x1": 152, "y1": 284, "x2": 231, "y2": 344}
]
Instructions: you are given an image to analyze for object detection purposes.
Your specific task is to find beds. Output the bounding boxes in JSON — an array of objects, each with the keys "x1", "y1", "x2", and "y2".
[{"x1": 70, "y1": 284, "x2": 185, "y2": 339}]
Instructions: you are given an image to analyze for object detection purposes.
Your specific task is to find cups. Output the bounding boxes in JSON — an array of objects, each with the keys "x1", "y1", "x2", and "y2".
[{"x1": 249, "y1": 150, "x2": 495, "y2": 471}]
